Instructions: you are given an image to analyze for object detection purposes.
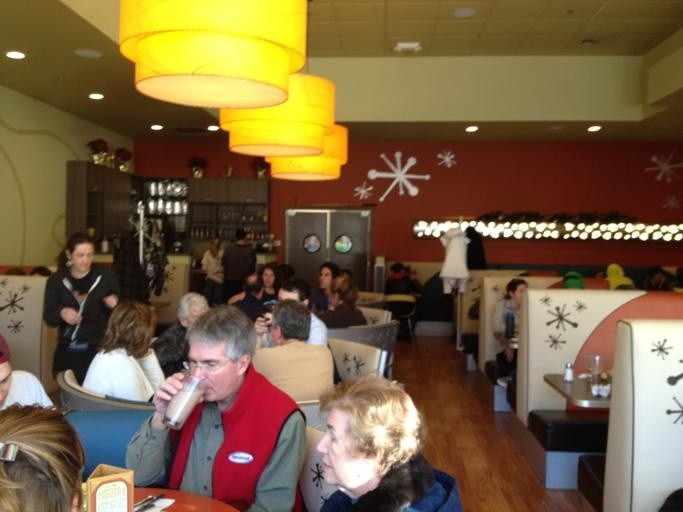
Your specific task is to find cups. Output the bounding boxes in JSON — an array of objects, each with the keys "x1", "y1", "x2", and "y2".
[{"x1": 161, "y1": 374, "x2": 207, "y2": 432}]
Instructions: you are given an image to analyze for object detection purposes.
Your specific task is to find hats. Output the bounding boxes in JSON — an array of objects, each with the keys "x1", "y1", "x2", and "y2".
[{"x1": 0, "y1": 336, "x2": 11, "y2": 364}]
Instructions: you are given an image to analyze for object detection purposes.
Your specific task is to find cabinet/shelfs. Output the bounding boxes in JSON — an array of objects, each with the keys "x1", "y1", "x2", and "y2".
[{"x1": 63, "y1": 159, "x2": 270, "y2": 245}]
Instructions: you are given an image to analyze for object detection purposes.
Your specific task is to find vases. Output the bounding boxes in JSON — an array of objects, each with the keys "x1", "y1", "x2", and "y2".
[{"x1": 92, "y1": 151, "x2": 106, "y2": 165}]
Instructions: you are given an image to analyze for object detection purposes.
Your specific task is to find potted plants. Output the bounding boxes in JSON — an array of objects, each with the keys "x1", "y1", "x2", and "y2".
[
  {"x1": 113, "y1": 148, "x2": 133, "y2": 173},
  {"x1": 186, "y1": 155, "x2": 208, "y2": 178}
]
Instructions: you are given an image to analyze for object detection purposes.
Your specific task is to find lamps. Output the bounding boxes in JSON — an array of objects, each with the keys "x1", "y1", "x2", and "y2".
[
  {"x1": 112, "y1": 1, "x2": 309, "y2": 110},
  {"x1": 263, "y1": 122, "x2": 350, "y2": 184},
  {"x1": 218, "y1": 73, "x2": 337, "y2": 159}
]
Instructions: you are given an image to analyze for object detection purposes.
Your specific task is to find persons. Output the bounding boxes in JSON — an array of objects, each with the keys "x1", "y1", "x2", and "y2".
[
  {"x1": 123, "y1": 306, "x2": 309, "y2": 511},
  {"x1": 41, "y1": 230, "x2": 121, "y2": 390},
  {"x1": 303, "y1": 370, "x2": 466, "y2": 511},
  {"x1": 74, "y1": 225, "x2": 425, "y2": 407},
  {"x1": 640, "y1": 265, "x2": 673, "y2": 291},
  {"x1": 490, "y1": 278, "x2": 530, "y2": 411},
  {"x1": 0, "y1": 399, "x2": 88, "y2": 512},
  {"x1": 588, "y1": 267, "x2": 607, "y2": 281},
  {"x1": 0, "y1": 333, "x2": 59, "y2": 415}
]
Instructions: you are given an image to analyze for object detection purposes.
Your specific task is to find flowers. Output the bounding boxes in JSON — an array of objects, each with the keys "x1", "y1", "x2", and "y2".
[{"x1": 86, "y1": 139, "x2": 108, "y2": 154}]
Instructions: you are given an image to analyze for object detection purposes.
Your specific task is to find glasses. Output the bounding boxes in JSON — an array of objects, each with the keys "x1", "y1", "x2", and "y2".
[{"x1": 182, "y1": 360, "x2": 229, "y2": 371}]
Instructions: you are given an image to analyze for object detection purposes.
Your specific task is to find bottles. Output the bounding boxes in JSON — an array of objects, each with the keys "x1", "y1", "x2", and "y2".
[
  {"x1": 585, "y1": 355, "x2": 601, "y2": 397},
  {"x1": 562, "y1": 363, "x2": 574, "y2": 383},
  {"x1": 505, "y1": 312, "x2": 513, "y2": 338}
]
